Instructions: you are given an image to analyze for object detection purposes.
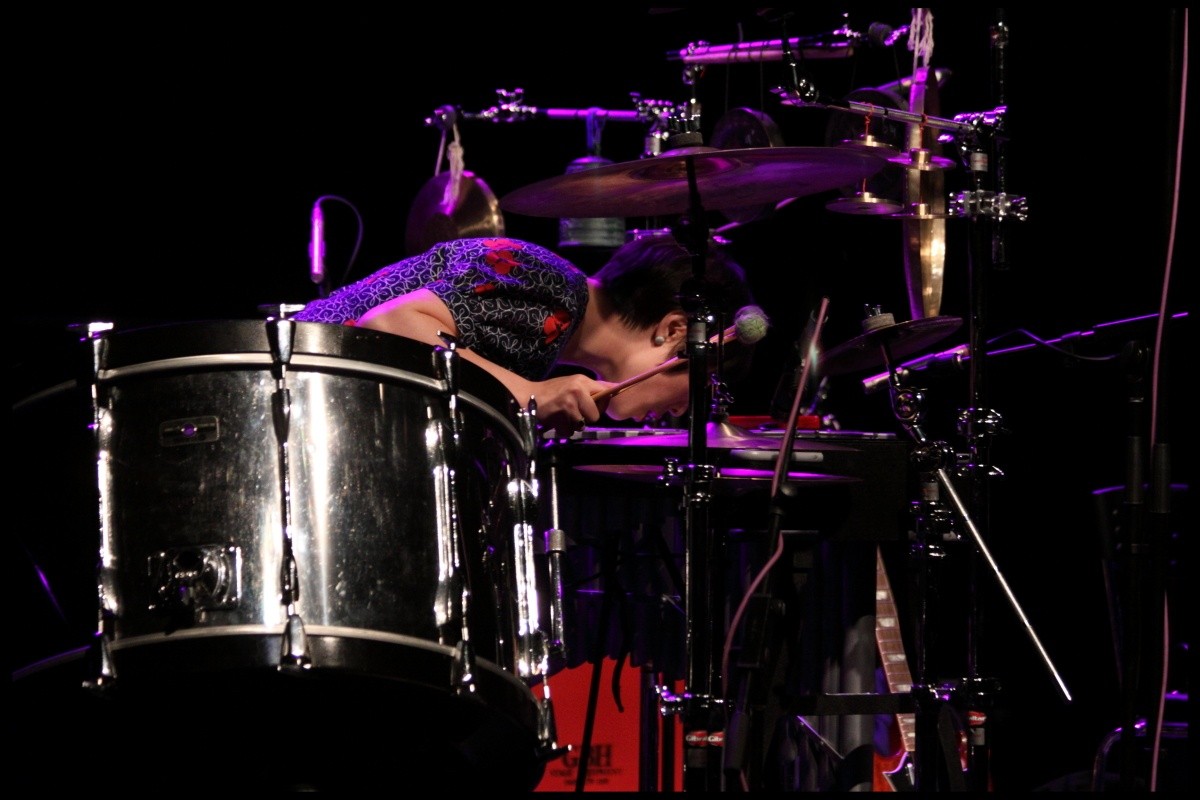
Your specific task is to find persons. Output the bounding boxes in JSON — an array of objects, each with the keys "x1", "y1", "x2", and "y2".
[
  {"x1": 711, "y1": 107, "x2": 920, "y2": 800},
  {"x1": 280, "y1": 234, "x2": 751, "y2": 439}
]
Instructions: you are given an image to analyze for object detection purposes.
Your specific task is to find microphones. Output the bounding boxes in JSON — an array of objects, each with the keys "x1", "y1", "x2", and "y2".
[
  {"x1": 309, "y1": 204, "x2": 328, "y2": 284},
  {"x1": 818, "y1": 342, "x2": 974, "y2": 416}
]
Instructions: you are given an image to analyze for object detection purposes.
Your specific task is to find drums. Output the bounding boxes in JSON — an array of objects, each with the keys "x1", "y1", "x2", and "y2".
[
  {"x1": 564, "y1": 420, "x2": 881, "y2": 669},
  {"x1": 0, "y1": 311, "x2": 566, "y2": 800}
]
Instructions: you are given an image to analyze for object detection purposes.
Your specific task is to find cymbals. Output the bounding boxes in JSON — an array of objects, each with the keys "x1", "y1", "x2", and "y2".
[
  {"x1": 823, "y1": 315, "x2": 964, "y2": 362},
  {"x1": 498, "y1": 138, "x2": 884, "y2": 223},
  {"x1": 570, "y1": 419, "x2": 869, "y2": 484}
]
